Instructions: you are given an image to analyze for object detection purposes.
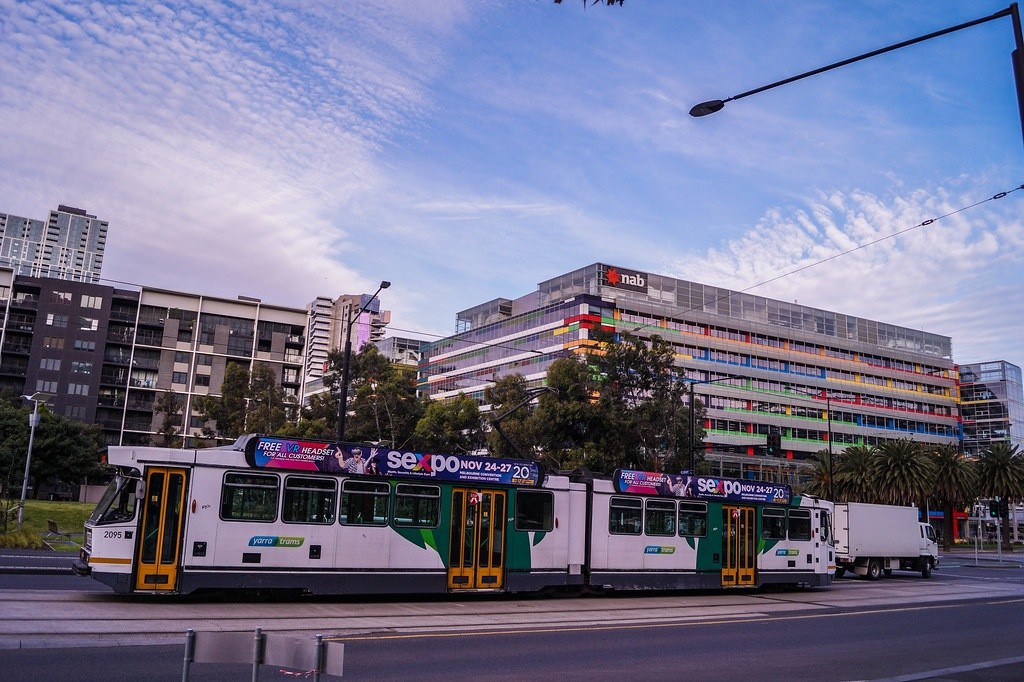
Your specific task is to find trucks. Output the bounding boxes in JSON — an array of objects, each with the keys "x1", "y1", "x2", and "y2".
[{"x1": 831, "y1": 501, "x2": 940, "y2": 576}]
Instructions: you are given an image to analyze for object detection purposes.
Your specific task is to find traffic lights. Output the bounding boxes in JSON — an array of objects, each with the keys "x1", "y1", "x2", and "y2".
[
  {"x1": 766, "y1": 433, "x2": 775, "y2": 454},
  {"x1": 990, "y1": 501, "x2": 998, "y2": 516}
]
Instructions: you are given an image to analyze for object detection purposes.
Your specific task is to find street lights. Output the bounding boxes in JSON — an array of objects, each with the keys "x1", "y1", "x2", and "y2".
[
  {"x1": 688, "y1": 373, "x2": 741, "y2": 474},
  {"x1": 687, "y1": 1, "x2": 1024, "y2": 135},
  {"x1": 779, "y1": 386, "x2": 869, "y2": 503},
  {"x1": 15, "y1": 390, "x2": 59, "y2": 522},
  {"x1": 334, "y1": 280, "x2": 392, "y2": 440}
]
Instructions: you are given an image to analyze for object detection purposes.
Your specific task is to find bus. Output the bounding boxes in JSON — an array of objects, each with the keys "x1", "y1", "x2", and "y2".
[{"x1": 70, "y1": 442, "x2": 839, "y2": 602}]
